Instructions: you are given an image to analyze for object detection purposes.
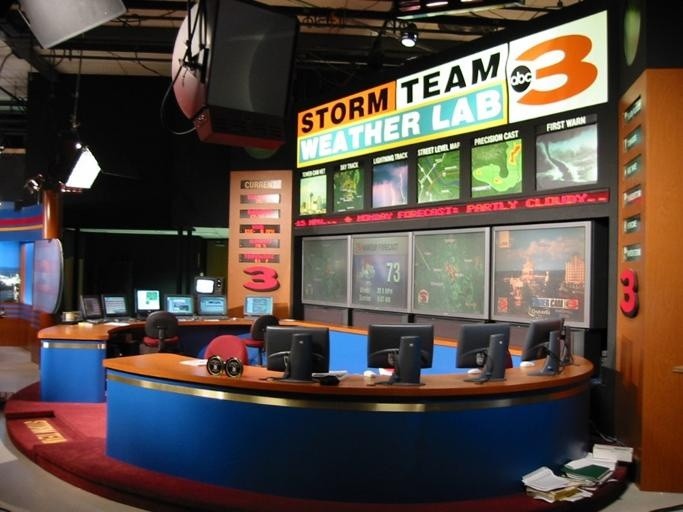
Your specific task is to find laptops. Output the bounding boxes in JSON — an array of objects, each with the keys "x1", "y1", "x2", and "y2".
[
  {"x1": 80, "y1": 295, "x2": 112, "y2": 324},
  {"x1": 164, "y1": 293, "x2": 199, "y2": 321},
  {"x1": 243, "y1": 296, "x2": 273, "y2": 321},
  {"x1": 101, "y1": 294, "x2": 134, "y2": 321},
  {"x1": 197, "y1": 294, "x2": 229, "y2": 320}
]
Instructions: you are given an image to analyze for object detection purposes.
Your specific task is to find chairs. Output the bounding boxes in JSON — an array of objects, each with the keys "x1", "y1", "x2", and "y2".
[
  {"x1": 244, "y1": 314, "x2": 279, "y2": 366},
  {"x1": 204, "y1": 334, "x2": 248, "y2": 365},
  {"x1": 505, "y1": 349, "x2": 512, "y2": 368},
  {"x1": 143, "y1": 311, "x2": 179, "y2": 353}
]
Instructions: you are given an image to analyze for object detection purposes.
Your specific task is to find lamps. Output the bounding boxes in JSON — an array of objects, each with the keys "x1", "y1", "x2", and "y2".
[{"x1": 398, "y1": 19, "x2": 419, "y2": 48}]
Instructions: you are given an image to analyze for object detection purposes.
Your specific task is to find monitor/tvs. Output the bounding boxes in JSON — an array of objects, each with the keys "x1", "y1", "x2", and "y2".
[
  {"x1": 367, "y1": 324, "x2": 434, "y2": 386},
  {"x1": 134, "y1": 288, "x2": 161, "y2": 313},
  {"x1": 333, "y1": 167, "x2": 364, "y2": 214},
  {"x1": 349, "y1": 231, "x2": 411, "y2": 314},
  {"x1": 471, "y1": 137, "x2": 522, "y2": 198},
  {"x1": 455, "y1": 324, "x2": 510, "y2": 382},
  {"x1": 536, "y1": 123, "x2": 597, "y2": 191},
  {"x1": 520, "y1": 319, "x2": 564, "y2": 376},
  {"x1": 265, "y1": 325, "x2": 330, "y2": 382},
  {"x1": 371, "y1": 157, "x2": 408, "y2": 208},
  {"x1": 417, "y1": 149, "x2": 460, "y2": 205},
  {"x1": 300, "y1": 175, "x2": 326, "y2": 215},
  {"x1": 411, "y1": 227, "x2": 490, "y2": 320},
  {"x1": 301, "y1": 235, "x2": 350, "y2": 308},
  {"x1": 194, "y1": 276, "x2": 224, "y2": 297},
  {"x1": 490, "y1": 220, "x2": 596, "y2": 328}
]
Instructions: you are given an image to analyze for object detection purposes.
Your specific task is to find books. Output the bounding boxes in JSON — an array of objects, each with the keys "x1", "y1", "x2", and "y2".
[
  {"x1": 179, "y1": 358, "x2": 208, "y2": 366},
  {"x1": 521, "y1": 442, "x2": 634, "y2": 504},
  {"x1": 104, "y1": 321, "x2": 131, "y2": 327}
]
